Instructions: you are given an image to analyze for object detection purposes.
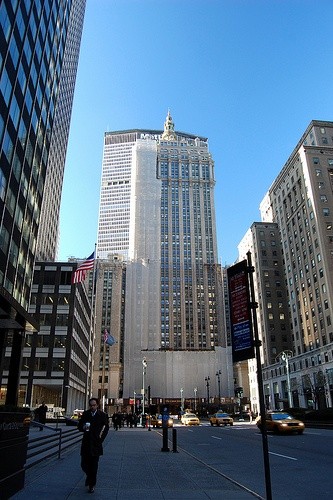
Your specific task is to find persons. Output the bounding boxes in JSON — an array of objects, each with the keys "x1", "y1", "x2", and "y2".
[
  {"x1": 78, "y1": 398, "x2": 110, "y2": 493},
  {"x1": 112, "y1": 410, "x2": 141, "y2": 431},
  {"x1": 38, "y1": 401, "x2": 47, "y2": 431}
]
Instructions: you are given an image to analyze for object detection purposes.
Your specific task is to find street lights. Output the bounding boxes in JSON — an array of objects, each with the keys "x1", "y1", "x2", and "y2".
[
  {"x1": 216, "y1": 370, "x2": 222, "y2": 405},
  {"x1": 204, "y1": 375, "x2": 211, "y2": 405},
  {"x1": 141, "y1": 354, "x2": 148, "y2": 426}
]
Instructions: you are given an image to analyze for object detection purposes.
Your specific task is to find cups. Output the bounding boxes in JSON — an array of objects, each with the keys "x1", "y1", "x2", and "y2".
[{"x1": 86, "y1": 422, "x2": 90, "y2": 431}]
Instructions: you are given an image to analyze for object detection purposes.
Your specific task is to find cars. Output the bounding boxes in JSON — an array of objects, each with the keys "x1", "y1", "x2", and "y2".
[
  {"x1": 181, "y1": 413, "x2": 200, "y2": 426},
  {"x1": 156, "y1": 414, "x2": 174, "y2": 428},
  {"x1": 210, "y1": 410, "x2": 234, "y2": 426},
  {"x1": 256, "y1": 411, "x2": 305, "y2": 436}
]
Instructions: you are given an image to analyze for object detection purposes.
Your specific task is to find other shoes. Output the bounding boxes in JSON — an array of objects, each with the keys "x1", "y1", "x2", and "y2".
[
  {"x1": 84, "y1": 476, "x2": 89, "y2": 487},
  {"x1": 89, "y1": 486, "x2": 95, "y2": 493}
]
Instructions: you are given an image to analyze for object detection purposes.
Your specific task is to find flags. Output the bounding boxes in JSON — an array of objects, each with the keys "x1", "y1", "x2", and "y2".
[
  {"x1": 104, "y1": 330, "x2": 117, "y2": 347},
  {"x1": 73, "y1": 253, "x2": 94, "y2": 284}
]
